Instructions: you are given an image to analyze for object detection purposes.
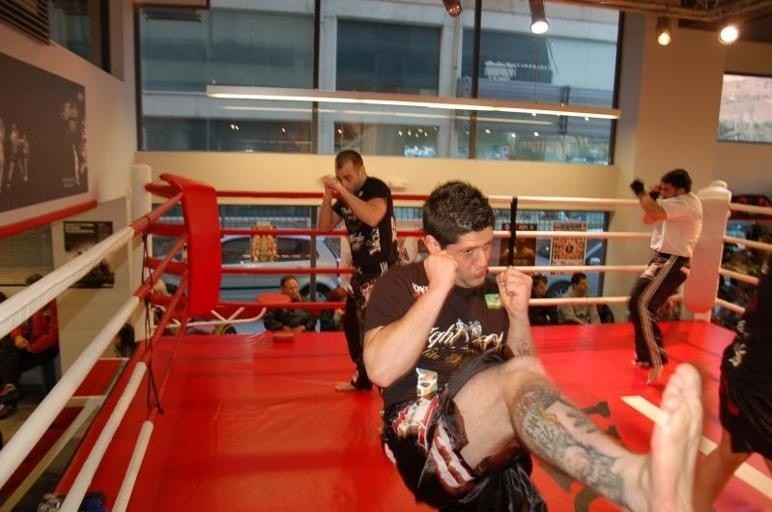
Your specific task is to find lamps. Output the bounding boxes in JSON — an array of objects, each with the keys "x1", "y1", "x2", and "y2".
[{"x1": 443, "y1": 0, "x2": 739, "y2": 46}]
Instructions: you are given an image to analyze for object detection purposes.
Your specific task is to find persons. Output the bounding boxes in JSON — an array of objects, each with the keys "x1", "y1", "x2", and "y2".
[
  {"x1": 556, "y1": 272, "x2": 603, "y2": 324},
  {"x1": 260, "y1": 274, "x2": 317, "y2": 332},
  {"x1": 623, "y1": 166, "x2": 706, "y2": 386},
  {"x1": 527, "y1": 274, "x2": 560, "y2": 326},
  {"x1": 687, "y1": 248, "x2": 772, "y2": 512},
  {"x1": 321, "y1": 285, "x2": 349, "y2": 331},
  {"x1": 0, "y1": 273, "x2": 59, "y2": 419},
  {"x1": 319, "y1": 148, "x2": 399, "y2": 392},
  {"x1": 361, "y1": 178, "x2": 709, "y2": 512}
]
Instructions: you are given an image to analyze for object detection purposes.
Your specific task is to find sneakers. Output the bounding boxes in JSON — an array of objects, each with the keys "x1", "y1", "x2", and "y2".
[
  {"x1": 1, "y1": 402, "x2": 17, "y2": 418},
  {"x1": 0, "y1": 384, "x2": 21, "y2": 400}
]
